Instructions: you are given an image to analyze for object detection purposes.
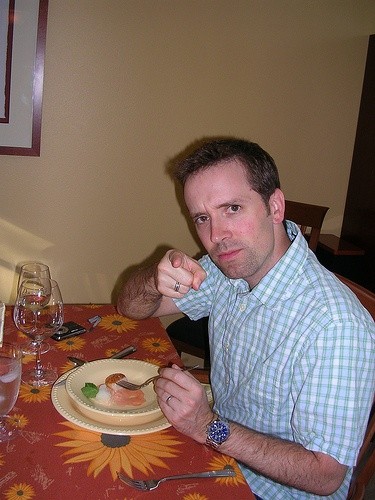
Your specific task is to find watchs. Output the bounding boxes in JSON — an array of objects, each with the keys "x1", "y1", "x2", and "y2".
[{"x1": 205, "y1": 416, "x2": 231, "y2": 451}]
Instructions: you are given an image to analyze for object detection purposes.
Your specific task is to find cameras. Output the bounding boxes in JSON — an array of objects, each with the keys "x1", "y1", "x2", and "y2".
[{"x1": 50, "y1": 321, "x2": 86, "y2": 340}]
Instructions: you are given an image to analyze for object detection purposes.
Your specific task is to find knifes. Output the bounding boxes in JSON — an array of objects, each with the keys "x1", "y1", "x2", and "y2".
[
  {"x1": 68, "y1": 356, "x2": 85, "y2": 366},
  {"x1": 54, "y1": 344, "x2": 138, "y2": 387}
]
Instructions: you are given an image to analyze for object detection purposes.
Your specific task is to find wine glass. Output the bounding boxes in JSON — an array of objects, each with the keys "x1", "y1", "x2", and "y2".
[
  {"x1": 13, "y1": 279, "x2": 64, "y2": 388},
  {"x1": 17, "y1": 264, "x2": 52, "y2": 354},
  {"x1": 0, "y1": 341, "x2": 22, "y2": 442}
]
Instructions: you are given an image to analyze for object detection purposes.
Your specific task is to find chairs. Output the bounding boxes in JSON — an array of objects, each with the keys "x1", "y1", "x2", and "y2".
[
  {"x1": 166, "y1": 201, "x2": 330, "y2": 355},
  {"x1": 336, "y1": 273, "x2": 375, "y2": 500}
]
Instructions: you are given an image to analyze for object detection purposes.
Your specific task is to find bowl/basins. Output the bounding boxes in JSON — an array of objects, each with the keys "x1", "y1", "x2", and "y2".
[{"x1": 66, "y1": 359, "x2": 164, "y2": 425}]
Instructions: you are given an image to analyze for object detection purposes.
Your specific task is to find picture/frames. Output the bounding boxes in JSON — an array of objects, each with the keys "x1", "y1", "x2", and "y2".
[{"x1": 0, "y1": 0, "x2": 49, "y2": 158}]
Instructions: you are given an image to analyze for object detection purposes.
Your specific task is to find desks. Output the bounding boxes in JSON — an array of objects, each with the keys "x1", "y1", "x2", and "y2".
[
  {"x1": 0, "y1": 304, "x2": 257, "y2": 500},
  {"x1": 305, "y1": 234, "x2": 364, "y2": 279}
]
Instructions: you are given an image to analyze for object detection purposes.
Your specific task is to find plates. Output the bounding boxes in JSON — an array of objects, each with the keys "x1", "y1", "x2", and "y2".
[{"x1": 51, "y1": 367, "x2": 173, "y2": 436}]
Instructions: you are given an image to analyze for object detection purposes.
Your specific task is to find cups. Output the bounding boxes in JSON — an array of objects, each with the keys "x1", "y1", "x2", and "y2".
[{"x1": 0, "y1": 302, "x2": 5, "y2": 347}]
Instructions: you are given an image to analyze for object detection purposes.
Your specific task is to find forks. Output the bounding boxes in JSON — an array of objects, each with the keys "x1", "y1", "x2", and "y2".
[
  {"x1": 115, "y1": 364, "x2": 200, "y2": 390},
  {"x1": 118, "y1": 468, "x2": 237, "y2": 491}
]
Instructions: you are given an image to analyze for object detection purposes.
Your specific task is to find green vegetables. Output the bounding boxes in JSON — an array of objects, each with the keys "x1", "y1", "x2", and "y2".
[{"x1": 81, "y1": 382, "x2": 99, "y2": 398}]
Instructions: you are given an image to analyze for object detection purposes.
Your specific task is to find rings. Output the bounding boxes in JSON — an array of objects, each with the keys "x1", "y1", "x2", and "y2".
[
  {"x1": 167, "y1": 395, "x2": 173, "y2": 405},
  {"x1": 174, "y1": 281, "x2": 180, "y2": 292}
]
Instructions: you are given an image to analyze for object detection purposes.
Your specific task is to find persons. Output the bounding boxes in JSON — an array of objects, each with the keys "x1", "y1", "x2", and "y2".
[{"x1": 117, "y1": 138, "x2": 375, "y2": 500}]
